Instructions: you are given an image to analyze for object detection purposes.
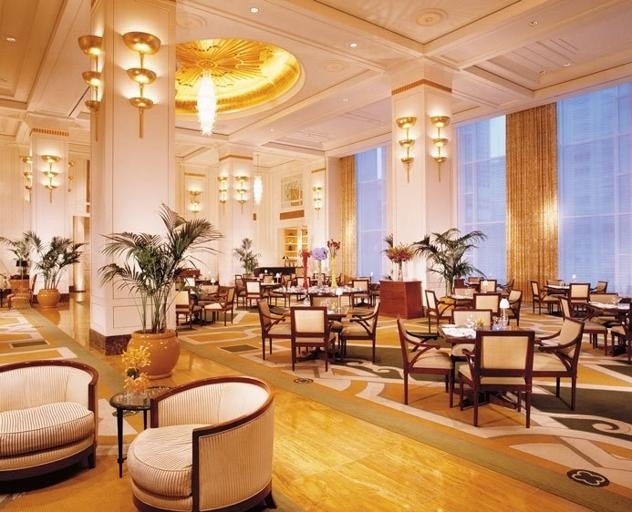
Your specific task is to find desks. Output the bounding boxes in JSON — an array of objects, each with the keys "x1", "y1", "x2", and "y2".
[{"x1": 111, "y1": 385, "x2": 175, "y2": 478}]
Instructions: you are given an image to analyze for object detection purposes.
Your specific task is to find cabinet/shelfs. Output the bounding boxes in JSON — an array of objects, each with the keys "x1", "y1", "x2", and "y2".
[{"x1": 283, "y1": 227, "x2": 309, "y2": 260}]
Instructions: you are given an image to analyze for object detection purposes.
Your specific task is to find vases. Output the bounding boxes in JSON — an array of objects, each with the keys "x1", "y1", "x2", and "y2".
[
  {"x1": 126, "y1": 385, "x2": 149, "y2": 400},
  {"x1": 302, "y1": 260, "x2": 309, "y2": 289},
  {"x1": 398, "y1": 261, "x2": 404, "y2": 283},
  {"x1": 318, "y1": 260, "x2": 324, "y2": 288},
  {"x1": 330, "y1": 256, "x2": 338, "y2": 289}
]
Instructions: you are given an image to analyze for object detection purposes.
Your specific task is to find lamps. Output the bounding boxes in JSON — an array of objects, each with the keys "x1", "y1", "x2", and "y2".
[
  {"x1": 312, "y1": 183, "x2": 322, "y2": 211},
  {"x1": 39, "y1": 153, "x2": 61, "y2": 202},
  {"x1": 122, "y1": 32, "x2": 161, "y2": 140},
  {"x1": 78, "y1": 34, "x2": 104, "y2": 143},
  {"x1": 21, "y1": 155, "x2": 33, "y2": 205},
  {"x1": 68, "y1": 158, "x2": 76, "y2": 195},
  {"x1": 395, "y1": 115, "x2": 417, "y2": 183},
  {"x1": 430, "y1": 115, "x2": 451, "y2": 182},
  {"x1": 187, "y1": 172, "x2": 251, "y2": 218}
]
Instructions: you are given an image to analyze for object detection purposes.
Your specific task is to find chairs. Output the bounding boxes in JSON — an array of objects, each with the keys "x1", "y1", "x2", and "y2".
[
  {"x1": 175, "y1": 266, "x2": 631, "y2": 430},
  {"x1": 0, "y1": 274, "x2": 8, "y2": 307},
  {"x1": 7, "y1": 273, "x2": 38, "y2": 310}
]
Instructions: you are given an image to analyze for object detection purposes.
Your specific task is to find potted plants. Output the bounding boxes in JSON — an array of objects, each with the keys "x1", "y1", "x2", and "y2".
[
  {"x1": 410, "y1": 226, "x2": 492, "y2": 310},
  {"x1": 96, "y1": 202, "x2": 225, "y2": 381},
  {"x1": 24, "y1": 228, "x2": 90, "y2": 309},
  {"x1": 0, "y1": 237, "x2": 31, "y2": 293}
]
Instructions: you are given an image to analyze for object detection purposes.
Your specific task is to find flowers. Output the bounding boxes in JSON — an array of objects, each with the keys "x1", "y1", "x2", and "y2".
[
  {"x1": 309, "y1": 248, "x2": 328, "y2": 271},
  {"x1": 381, "y1": 242, "x2": 417, "y2": 269},
  {"x1": 327, "y1": 239, "x2": 342, "y2": 259},
  {"x1": 118, "y1": 343, "x2": 154, "y2": 392},
  {"x1": 300, "y1": 248, "x2": 311, "y2": 267}
]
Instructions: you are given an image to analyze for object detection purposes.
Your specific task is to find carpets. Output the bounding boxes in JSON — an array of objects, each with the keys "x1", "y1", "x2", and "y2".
[
  {"x1": 178, "y1": 295, "x2": 630, "y2": 511},
  {"x1": 1, "y1": 304, "x2": 304, "y2": 511}
]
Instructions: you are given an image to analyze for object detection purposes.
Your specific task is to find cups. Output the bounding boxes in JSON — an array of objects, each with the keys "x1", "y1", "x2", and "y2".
[
  {"x1": 464, "y1": 313, "x2": 476, "y2": 328},
  {"x1": 495, "y1": 316, "x2": 509, "y2": 330},
  {"x1": 510, "y1": 319, "x2": 517, "y2": 331},
  {"x1": 320, "y1": 300, "x2": 342, "y2": 313},
  {"x1": 277, "y1": 285, "x2": 360, "y2": 296}
]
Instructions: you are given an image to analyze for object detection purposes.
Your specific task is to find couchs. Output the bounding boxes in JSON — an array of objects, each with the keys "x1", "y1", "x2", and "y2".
[
  {"x1": 125, "y1": 374, "x2": 278, "y2": 511},
  {"x1": 1, "y1": 359, "x2": 100, "y2": 490}
]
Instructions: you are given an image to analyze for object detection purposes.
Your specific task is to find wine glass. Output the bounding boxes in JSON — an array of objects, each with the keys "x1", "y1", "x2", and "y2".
[
  {"x1": 606, "y1": 295, "x2": 623, "y2": 306},
  {"x1": 210, "y1": 275, "x2": 215, "y2": 286}
]
